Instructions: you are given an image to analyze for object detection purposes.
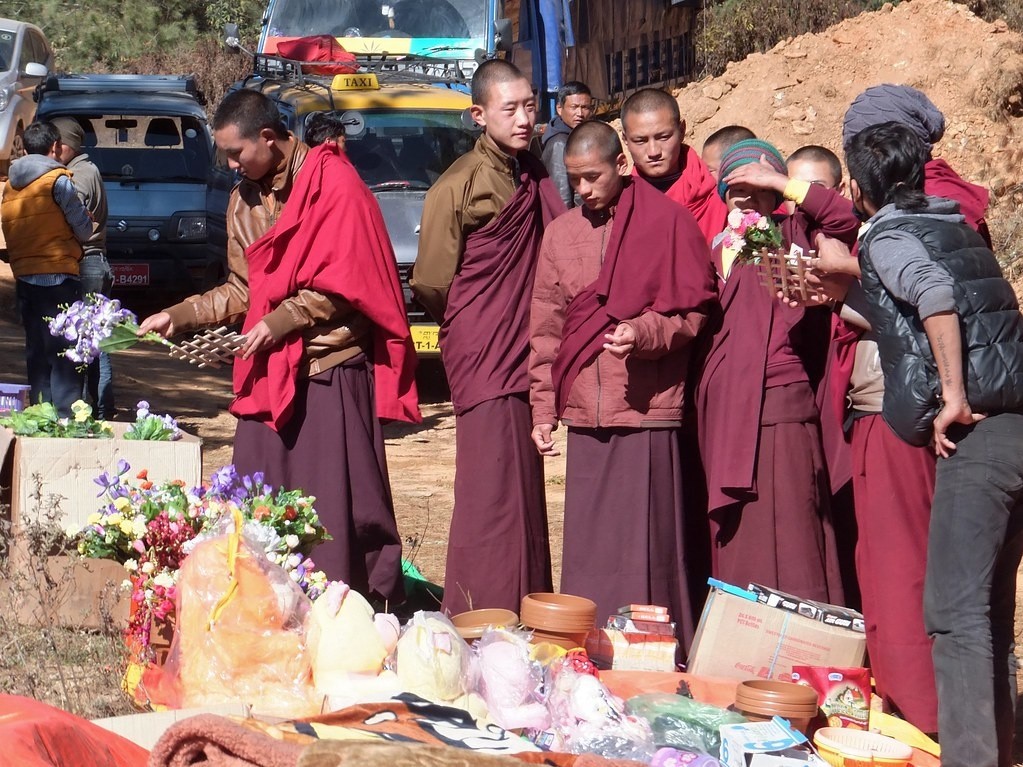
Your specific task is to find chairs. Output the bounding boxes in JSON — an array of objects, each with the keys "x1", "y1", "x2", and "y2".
[
  {"x1": 145, "y1": 117, "x2": 185, "y2": 172},
  {"x1": 76, "y1": 119, "x2": 102, "y2": 170},
  {"x1": 397, "y1": 135, "x2": 439, "y2": 177}
]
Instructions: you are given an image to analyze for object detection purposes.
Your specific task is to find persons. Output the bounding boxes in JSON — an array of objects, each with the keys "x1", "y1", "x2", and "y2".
[
  {"x1": 414, "y1": 59, "x2": 570, "y2": 623},
  {"x1": 1, "y1": 120, "x2": 95, "y2": 418},
  {"x1": 539, "y1": 81, "x2": 592, "y2": 211},
  {"x1": 305, "y1": 118, "x2": 347, "y2": 154},
  {"x1": 620, "y1": 87, "x2": 728, "y2": 250},
  {"x1": 777, "y1": 85, "x2": 994, "y2": 741},
  {"x1": 690, "y1": 139, "x2": 858, "y2": 606},
  {"x1": 702, "y1": 125, "x2": 757, "y2": 186},
  {"x1": 527, "y1": 119, "x2": 716, "y2": 669},
  {"x1": 807, "y1": 121, "x2": 1022, "y2": 767},
  {"x1": 136, "y1": 88, "x2": 423, "y2": 605},
  {"x1": 52, "y1": 118, "x2": 115, "y2": 420},
  {"x1": 787, "y1": 146, "x2": 846, "y2": 197}
]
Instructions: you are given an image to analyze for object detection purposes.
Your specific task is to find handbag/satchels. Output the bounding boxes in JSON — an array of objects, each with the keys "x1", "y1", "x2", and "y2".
[{"x1": 276, "y1": 35, "x2": 360, "y2": 75}]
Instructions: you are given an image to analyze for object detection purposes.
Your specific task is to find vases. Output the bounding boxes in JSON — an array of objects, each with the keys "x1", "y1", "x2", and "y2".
[
  {"x1": 452, "y1": 606, "x2": 520, "y2": 644},
  {"x1": 734, "y1": 677, "x2": 819, "y2": 735},
  {"x1": 521, "y1": 593, "x2": 597, "y2": 651}
]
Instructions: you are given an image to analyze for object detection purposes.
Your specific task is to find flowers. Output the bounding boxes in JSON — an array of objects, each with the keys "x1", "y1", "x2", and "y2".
[
  {"x1": 721, "y1": 208, "x2": 784, "y2": 281},
  {"x1": 2, "y1": 397, "x2": 181, "y2": 442},
  {"x1": 42, "y1": 293, "x2": 173, "y2": 373},
  {"x1": 75, "y1": 462, "x2": 335, "y2": 656}
]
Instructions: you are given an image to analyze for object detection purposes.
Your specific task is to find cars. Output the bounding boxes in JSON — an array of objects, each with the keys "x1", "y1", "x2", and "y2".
[{"x1": 0, "y1": 17, "x2": 56, "y2": 177}]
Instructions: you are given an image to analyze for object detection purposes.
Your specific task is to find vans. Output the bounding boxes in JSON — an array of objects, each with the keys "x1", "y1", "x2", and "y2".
[
  {"x1": 28, "y1": 69, "x2": 235, "y2": 311},
  {"x1": 237, "y1": 47, "x2": 481, "y2": 360}
]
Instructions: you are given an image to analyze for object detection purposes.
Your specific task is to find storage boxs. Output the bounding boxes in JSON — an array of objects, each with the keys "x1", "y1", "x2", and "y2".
[
  {"x1": 689, "y1": 587, "x2": 869, "y2": 683},
  {"x1": 0, "y1": 420, "x2": 204, "y2": 567},
  {"x1": 719, "y1": 715, "x2": 831, "y2": 767}
]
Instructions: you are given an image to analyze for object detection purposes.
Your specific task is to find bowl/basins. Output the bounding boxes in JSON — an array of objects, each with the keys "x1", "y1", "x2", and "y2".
[{"x1": 811, "y1": 724, "x2": 914, "y2": 767}]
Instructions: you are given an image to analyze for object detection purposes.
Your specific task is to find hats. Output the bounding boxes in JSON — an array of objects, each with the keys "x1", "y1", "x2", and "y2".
[
  {"x1": 49, "y1": 116, "x2": 85, "y2": 152},
  {"x1": 843, "y1": 83, "x2": 945, "y2": 153},
  {"x1": 717, "y1": 139, "x2": 788, "y2": 213}
]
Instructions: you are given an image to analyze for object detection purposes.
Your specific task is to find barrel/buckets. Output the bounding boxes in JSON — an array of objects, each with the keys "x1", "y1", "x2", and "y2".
[
  {"x1": 450, "y1": 607, "x2": 524, "y2": 642},
  {"x1": 735, "y1": 680, "x2": 819, "y2": 740},
  {"x1": 520, "y1": 593, "x2": 598, "y2": 654}
]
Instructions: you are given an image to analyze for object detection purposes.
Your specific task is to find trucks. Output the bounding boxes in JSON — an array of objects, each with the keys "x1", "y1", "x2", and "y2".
[{"x1": 222, "y1": 0, "x2": 513, "y2": 78}]
